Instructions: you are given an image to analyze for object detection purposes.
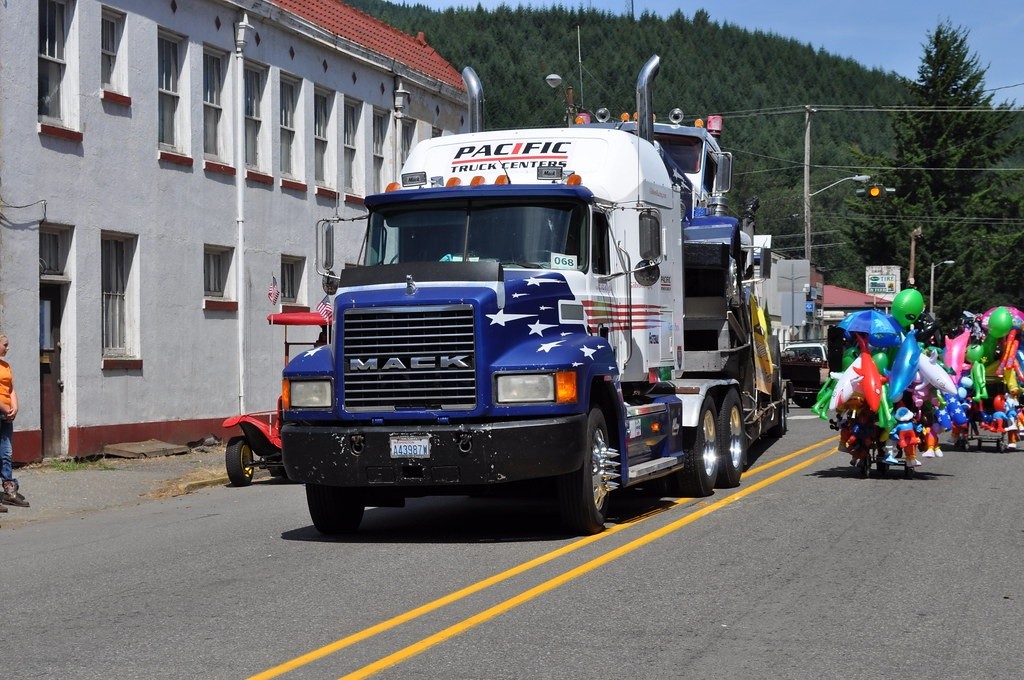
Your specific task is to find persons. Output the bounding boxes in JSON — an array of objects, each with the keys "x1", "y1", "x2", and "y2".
[{"x1": 0, "y1": 334, "x2": 29, "y2": 512}]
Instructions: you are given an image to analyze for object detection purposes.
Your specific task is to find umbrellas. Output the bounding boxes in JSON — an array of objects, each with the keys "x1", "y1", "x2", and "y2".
[
  {"x1": 836, "y1": 309, "x2": 905, "y2": 335},
  {"x1": 981, "y1": 306, "x2": 1024, "y2": 330}
]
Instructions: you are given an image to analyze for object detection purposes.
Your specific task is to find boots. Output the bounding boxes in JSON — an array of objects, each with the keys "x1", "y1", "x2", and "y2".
[
  {"x1": 0, "y1": 503, "x2": 8, "y2": 512},
  {"x1": 2, "y1": 479, "x2": 29, "y2": 506}
]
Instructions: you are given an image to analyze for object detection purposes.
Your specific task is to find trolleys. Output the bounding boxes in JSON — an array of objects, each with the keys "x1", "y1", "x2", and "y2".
[
  {"x1": 962, "y1": 372, "x2": 1010, "y2": 453},
  {"x1": 862, "y1": 442, "x2": 914, "y2": 479}
]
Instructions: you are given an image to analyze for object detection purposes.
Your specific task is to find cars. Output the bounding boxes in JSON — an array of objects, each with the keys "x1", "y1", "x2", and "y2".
[{"x1": 781, "y1": 340, "x2": 828, "y2": 363}]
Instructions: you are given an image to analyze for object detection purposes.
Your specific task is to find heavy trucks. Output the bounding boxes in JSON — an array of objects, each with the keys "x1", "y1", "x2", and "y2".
[{"x1": 281, "y1": 54, "x2": 797, "y2": 534}]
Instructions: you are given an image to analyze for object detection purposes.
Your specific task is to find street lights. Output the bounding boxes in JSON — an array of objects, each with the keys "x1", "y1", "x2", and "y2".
[
  {"x1": 802, "y1": 174, "x2": 873, "y2": 259},
  {"x1": 929, "y1": 260, "x2": 955, "y2": 315}
]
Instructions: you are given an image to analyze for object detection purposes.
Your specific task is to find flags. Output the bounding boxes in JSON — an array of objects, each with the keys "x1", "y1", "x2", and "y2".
[
  {"x1": 267, "y1": 276, "x2": 280, "y2": 306},
  {"x1": 316, "y1": 295, "x2": 334, "y2": 322}
]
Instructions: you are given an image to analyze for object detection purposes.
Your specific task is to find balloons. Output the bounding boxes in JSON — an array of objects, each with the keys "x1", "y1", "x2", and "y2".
[{"x1": 811, "y1": 289, "x2": 1024, "y2": 467}]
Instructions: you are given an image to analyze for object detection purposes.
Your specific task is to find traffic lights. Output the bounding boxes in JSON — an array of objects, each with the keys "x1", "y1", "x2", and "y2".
[
  {"x1": 868, "y1": 182, "x2": 885, "y2": 200},
  {"x1": 856, "y1": 185, "x2": 868, "y2": 198},
  {"x1": 881, "y1": 185, "x2": 896, "y2": 201}
]
